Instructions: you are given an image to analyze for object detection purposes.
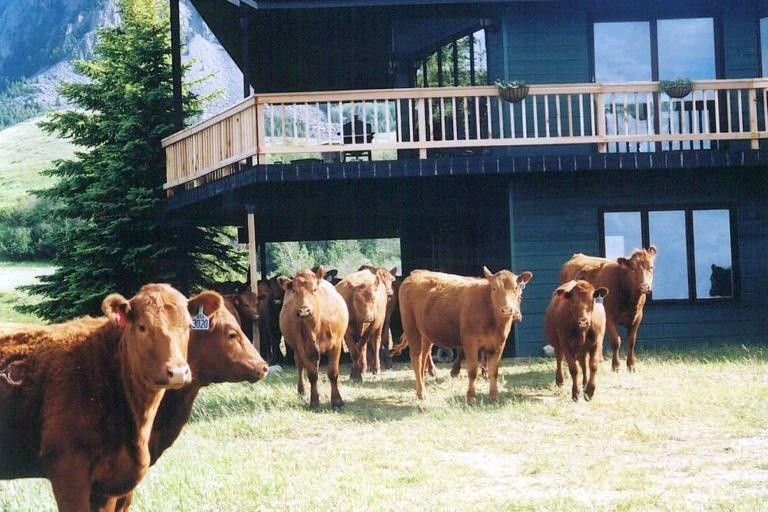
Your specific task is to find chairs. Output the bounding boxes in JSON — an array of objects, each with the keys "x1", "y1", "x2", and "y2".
[{"x1": 337, "y1": 115, "x2": 373, "y2": 161}]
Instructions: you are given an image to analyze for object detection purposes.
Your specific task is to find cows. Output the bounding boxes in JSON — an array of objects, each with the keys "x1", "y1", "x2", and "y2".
[
  {"x1": 0, "y1": 282, "x2": 225, "y2": 512},
  {"x1": 546, "y1": 280, "x2": 609, "y2": 403},
  {"x1": 200, "y1": 264, "x2": 403, "y2": 413},
  {"x1": 558, "y1": 246, "x2": 658, "y2": 374},
  {"x1": 388, "y1": 265, "x2": 533, "y2": 407},
  {"x1": 42, "y1": 290, "x2": 269, "y2": 512}
]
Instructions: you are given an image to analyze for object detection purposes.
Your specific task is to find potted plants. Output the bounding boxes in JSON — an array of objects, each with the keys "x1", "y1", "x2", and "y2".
[
  {"x1": 657, "y1": 78, "x2": 697, "y2": 98},
  {"x1": 494, "y1": 79, "x2": 530, "y2": 103}
]
